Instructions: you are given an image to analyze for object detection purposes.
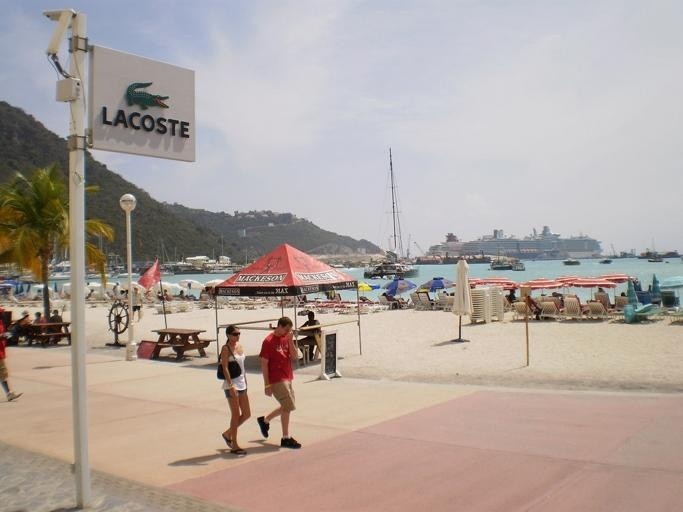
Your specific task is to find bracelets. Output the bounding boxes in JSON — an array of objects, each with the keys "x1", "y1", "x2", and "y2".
[
  {"x1": 228, "y1": 383, "x2": 235, "y2": 389},
  {"x1": 264, "y1": 383, "x2": 271, "y2": 389}
]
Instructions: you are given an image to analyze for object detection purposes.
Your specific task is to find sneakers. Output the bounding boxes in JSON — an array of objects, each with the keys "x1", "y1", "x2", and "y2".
[
  {"x1": 8, "y1": 392, "x2": 24, "y2": 402},
  {"x1": 257, "y1": 416, "x2": 270, "y2": 438},
  {"x1": 280, "y1": 436, "x2": 301, "y2": 449}
]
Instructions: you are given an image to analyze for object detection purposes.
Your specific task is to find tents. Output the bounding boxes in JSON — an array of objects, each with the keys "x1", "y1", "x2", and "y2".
[{"x1": 214, "y1": 242, "x2": 361, "y2": 356}]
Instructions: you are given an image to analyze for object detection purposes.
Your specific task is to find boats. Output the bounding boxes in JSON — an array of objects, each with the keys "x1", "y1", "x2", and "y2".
[
  {"x1": 597, "y1": 236, "x2": 682, "y2": 264},
  {"x1": 485, "y1": 243, "x2": 525, "y2": 272},
  {"x1": 559, "y1": 258, "x2": 579, "y2": 265},
  {"x1": 0, "y1": 238, "x2": 251, "y2": 295}
]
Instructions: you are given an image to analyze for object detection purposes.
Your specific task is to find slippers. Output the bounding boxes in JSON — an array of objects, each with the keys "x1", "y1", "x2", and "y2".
[
  {"x1": 230, "y1": 448, "x2": 247, "y2": 455},
  {"x1": 222, "y1": 433, "x2": 233, "y2": 448}
]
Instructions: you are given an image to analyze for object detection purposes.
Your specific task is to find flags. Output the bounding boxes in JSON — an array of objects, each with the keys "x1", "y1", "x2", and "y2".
[{"x1": 138, "y1": 259, "x2": 160, "y2": 291}]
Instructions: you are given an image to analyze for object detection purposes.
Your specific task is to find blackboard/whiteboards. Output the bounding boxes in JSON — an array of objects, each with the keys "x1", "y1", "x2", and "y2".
[{"x1": 322, "y1": 332, "x2": 337, "y2": 375}]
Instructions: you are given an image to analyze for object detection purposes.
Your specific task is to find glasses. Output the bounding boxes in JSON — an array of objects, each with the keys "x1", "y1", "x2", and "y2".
[{"x1": 229, "y1": 332, "x2": 241, "y2": 336}]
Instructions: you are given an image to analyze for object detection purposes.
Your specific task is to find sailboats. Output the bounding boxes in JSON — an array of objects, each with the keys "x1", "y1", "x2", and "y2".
[{"x1": 360, "y1": 147, "x2": 420, "y2": 278}]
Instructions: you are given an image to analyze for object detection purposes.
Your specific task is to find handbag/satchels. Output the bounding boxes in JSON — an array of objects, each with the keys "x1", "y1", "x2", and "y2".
[{"x1": 217, "y1": 361, "x2": 241, "y2": 379}]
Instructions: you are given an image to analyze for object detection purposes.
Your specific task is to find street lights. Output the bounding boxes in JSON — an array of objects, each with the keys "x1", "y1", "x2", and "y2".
[{"x1": 118, "y1": 193, "x2": 140, "y2": 361}]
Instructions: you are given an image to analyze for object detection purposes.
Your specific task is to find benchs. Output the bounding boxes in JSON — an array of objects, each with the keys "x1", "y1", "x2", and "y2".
[
  {"x1": 141, "y1": 328, "x2": 219, "y2": 362},
  {"x1": 6, "y1": 312, "x2": 73, "y2": 350}
]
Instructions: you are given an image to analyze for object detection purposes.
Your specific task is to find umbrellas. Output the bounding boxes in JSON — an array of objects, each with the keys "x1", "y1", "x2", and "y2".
[
  {"x1": 347, "y1": 272, "x2": 637, "y2": 294},
  {"x1": 627, "y1": 279, "x2": 638, "y2": 310},
  {"x1": 453, "y1": 259, "x2": 475, "y2": 340},
  {"x1": 652, "y1": 273, "x2": 661, "y2": 293},
  {"x1": 660, "y1": 276, "x2": 683, "y2": 298}
]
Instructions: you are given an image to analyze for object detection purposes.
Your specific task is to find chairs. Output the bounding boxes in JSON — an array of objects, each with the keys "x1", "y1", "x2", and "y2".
[{"x1": 275, "y1": 324, "x2": 325, "y2": 366}]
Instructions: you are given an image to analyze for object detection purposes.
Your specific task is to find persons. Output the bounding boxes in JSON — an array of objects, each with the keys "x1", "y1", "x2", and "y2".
[
  {"x1": 13, "y1": 282, "x2": 129, "y2": 300},
  {"x1": 157, "y1": 287, "x2": 212, "y2": 301},
  {"x1": 256, "y1": 316, "x2": 303, "y2": 450},
  {"x1": 3, "y1": 310, "x2": 63, "y2": 348},
  {"x1": 0, "y1": 307, "x2": 24, "y2": 403},
  {"x1": 130, "y1": 286, "x2": 144, "y2": 324},
  {"x1": 504, "y1": 283, "x2": 652, "y2": 304},
  {"x1": 525, "y1": 295, "x2": 542, "y2": 321},
  {"x1": 295, "y1": 311, "x2": 323, "y2": 362},
  {"x1": 293, "y1": 290, "x2": 449, "y2": 309},
  {"x1": 217, "y1": 325, "x2": 252, "y2": 455}
]
